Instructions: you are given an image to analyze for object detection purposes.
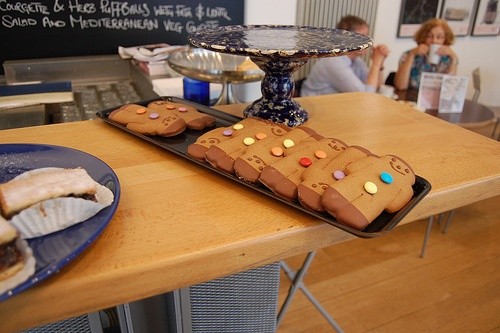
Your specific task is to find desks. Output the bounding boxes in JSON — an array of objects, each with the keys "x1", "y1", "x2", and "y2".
[
  {"x1": 0, "y1": 92, "x2": 500, "y2": 333},
  {"x1": 412, "y1": 100, "x2": 497, "y2": 225}
]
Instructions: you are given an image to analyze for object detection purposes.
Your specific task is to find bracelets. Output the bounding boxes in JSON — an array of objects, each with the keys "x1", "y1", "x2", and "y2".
[{"x1": 380, "y1": 66, "x2": 384, "y2": 71}]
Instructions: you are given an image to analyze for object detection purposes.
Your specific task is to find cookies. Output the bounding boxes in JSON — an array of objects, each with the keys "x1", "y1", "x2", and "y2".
[
  {"x1": 148, "y1": 101, "x2": 216, "y2": 130},
  {"x1": 188, "y1": 116, "x2": 416, "y2": 230},
  {"x1": 109, "y1": 103, "x2": 186, "y2": 136}
]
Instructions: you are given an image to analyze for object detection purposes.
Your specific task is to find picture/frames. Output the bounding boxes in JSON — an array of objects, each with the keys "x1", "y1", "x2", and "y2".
[{"x1": 397, "y1": 0, "x2": 500, "y2": 38}]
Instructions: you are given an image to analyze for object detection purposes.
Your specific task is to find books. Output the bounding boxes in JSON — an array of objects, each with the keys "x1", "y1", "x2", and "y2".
[{"x1": 417, "y1": 72, "x2": 468, "y2": 113}]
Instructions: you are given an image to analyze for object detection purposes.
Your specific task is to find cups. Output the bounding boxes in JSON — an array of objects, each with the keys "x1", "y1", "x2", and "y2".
[
  {"x1": 183, "y1": 76, "x2": 211, "y2": 107},
  {"x1": 378, "y1": 84, "x2": 394, "y2": 98},
  {"x1": 423, "y1": 44, "x2": 443, "y2": 65}
]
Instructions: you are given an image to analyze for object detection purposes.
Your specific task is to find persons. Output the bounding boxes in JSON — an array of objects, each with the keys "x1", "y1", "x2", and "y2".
[
  {"x1": 395, "y1": 19, "x2": 458, "y2": 90},
  {"x1": 301, "y1": 16, "x2": 390, "y2": 97}
]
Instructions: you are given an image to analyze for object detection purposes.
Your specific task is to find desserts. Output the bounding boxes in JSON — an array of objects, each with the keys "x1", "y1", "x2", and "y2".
[{"x1": 0, "y1": 166, "x2": 114, "y2": 296}]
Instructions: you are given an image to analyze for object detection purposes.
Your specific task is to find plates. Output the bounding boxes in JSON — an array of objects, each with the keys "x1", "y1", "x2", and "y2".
[
  {"x1": 92, "y1": 96, "x2": 432, "y2": 239},
  {"x1": 0, "y1": 143, "x2": 121, "y2": 301}
]
